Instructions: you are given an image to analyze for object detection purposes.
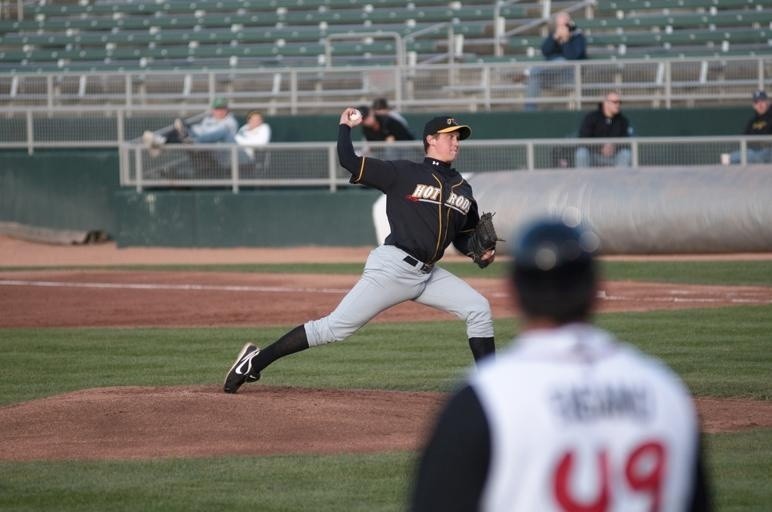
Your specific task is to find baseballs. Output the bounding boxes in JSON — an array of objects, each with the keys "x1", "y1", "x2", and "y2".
[{"x1": 349, "y1": 109, "x2": 361, "y2": 122}]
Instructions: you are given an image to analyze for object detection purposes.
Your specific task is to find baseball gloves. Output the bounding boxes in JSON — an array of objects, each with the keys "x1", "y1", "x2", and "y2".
[{"x1": 467, "y1": 212, "x2": 497, "y2": 269}]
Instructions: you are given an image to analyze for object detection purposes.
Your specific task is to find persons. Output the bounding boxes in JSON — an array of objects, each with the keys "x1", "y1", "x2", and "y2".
[
  {"x1": 574, "y1": 91, "x2": 631, "y2": 168},
  {"x1": 224, "y1": 106, "x2": 496, "y2": 394},
  {"x1": 721, "y1": 91, "x2": 772, "y2": 165},
  {"x1": 411, "y1": 221, "x2": 714, "y2": 512},
  {"x1": 523, "y1": 10, "x2": 587, "y2": 112},
  {"x1": 356, "y1": 98, "x2": 416, "y2": 160},
  {"x1": 142, "y1": 97, "x2": 272, "y2": 177}
]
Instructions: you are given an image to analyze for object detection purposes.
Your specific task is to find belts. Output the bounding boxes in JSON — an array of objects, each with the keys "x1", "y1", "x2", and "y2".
[{"x1": 403, "y1": 255, "x2": 433, "y2": 275}]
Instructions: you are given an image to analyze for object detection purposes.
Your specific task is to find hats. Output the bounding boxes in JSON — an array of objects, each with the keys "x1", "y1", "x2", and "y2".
[
  {"x1": 422, "y1": 116, "x2": 471, "y2": 142},
  {"x1": 212, "y1": 98, "x2": 229, "y2": 109},
  {"x1": 753, "y1": 91, "x2": 766, "y2": 102}
]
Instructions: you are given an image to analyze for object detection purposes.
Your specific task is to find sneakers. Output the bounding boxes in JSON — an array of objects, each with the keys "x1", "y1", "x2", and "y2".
[
  {"x1": 223, "y1": 342, "x2": 261, "y2": 394},
  {"x1": 143, "y1": 131, "x2": 166, "y2": 158}
]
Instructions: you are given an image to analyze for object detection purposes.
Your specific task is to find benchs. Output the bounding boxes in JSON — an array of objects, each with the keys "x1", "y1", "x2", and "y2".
[{"x1": 0, "y1": 0, "x2": 772, "y2": 104}]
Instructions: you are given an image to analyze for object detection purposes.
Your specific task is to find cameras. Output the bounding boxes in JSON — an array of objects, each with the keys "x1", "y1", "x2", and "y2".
[{"x1": 565, "y1": 22, "x2": 577, "y2": 31}]
